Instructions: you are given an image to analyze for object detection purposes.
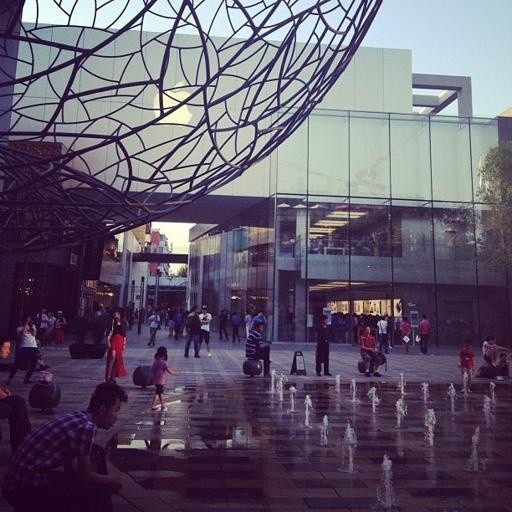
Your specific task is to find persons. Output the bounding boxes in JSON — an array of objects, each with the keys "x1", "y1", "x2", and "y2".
[
  {"x1": 0, "y1": 380, "x2": 129, "y2": 512},
  {"x1": 0, "y1": 334, "x2": 32, "y2": 455},
  {"x1": 9, "y1": 308, "x2": 67, "y2": 384}
]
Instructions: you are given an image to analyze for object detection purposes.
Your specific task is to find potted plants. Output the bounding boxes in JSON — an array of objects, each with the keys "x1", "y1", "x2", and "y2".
[
  {"x1": 89, "y1": 314, "x2": 106, "y2": 360},
  {"x1": 68, "y1": 317, "x2": 89, "y2": 360}
]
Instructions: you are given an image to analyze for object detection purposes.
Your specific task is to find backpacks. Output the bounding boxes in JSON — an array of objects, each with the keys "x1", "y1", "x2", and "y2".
[{"x1": 189, "y1": 316, "x2": 201, "y2": 336}]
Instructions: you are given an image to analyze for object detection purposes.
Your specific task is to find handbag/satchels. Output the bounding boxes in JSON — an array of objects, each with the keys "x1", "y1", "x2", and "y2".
[{"x1": 101, "y1": 328, "x2": 114, "y2": 345}]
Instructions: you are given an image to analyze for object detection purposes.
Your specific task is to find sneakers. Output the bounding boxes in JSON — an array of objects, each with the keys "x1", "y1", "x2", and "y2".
[
  {"x1": 161, "y1": 406, "x2": 168, "y2": 411},
  {"x1": 207, "y1": 353, "x2": 212, "y2": 357},
  {"x1": 194, "y1": 354, "x2": 200, "y2": 358},
  {"x1": 184, "y1": 354, "x2": 189, "y2": 358},
  {"x1": 152, "y1": 406, "x2": 159, "y2": 410}
]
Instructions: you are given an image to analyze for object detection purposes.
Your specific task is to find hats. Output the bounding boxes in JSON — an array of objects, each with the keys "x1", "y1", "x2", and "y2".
[{"x1": 202, "y1": 304, "x2": 208, "y2": 310}]
